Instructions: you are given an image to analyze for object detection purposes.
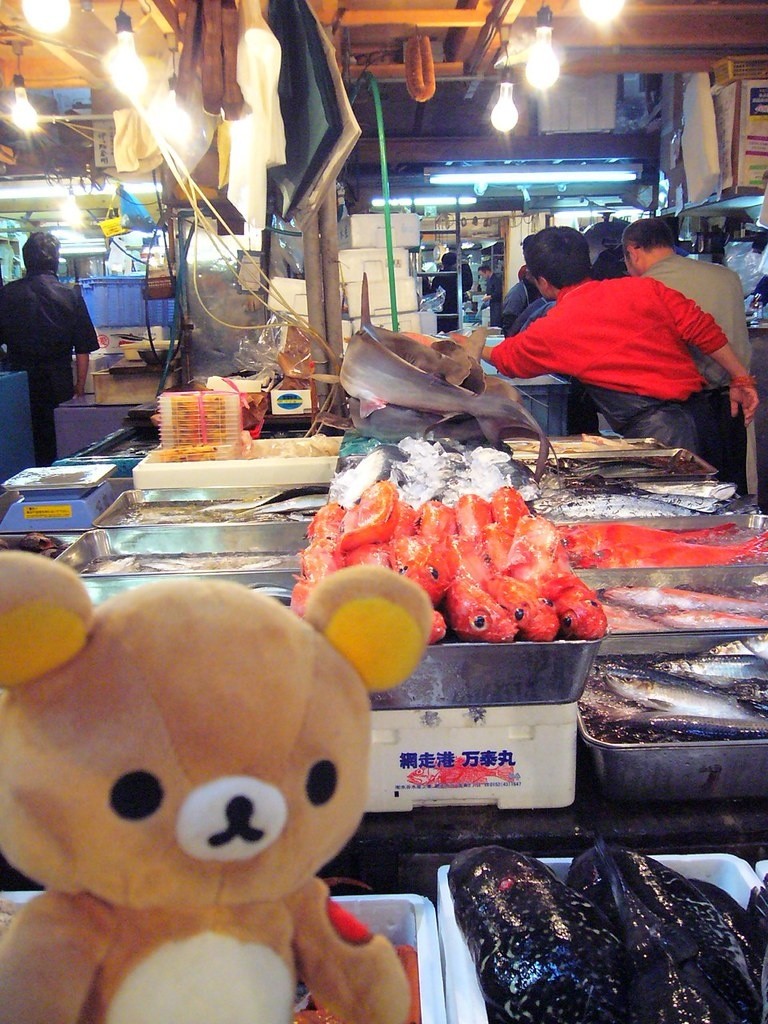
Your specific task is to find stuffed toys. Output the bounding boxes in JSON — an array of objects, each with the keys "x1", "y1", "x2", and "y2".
[{"x1": 1, "y1": 548, "x2": 435, "y2": 1024}]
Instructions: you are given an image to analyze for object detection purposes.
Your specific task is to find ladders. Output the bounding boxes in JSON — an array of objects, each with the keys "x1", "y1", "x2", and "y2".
[{"x1": 412, "y1": 212, "x2": 463, "y2": 332}]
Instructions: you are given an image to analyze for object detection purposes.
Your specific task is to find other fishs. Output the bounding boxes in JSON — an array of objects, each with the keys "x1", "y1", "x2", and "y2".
[
  {"x1": 533, "y1": 479, "x2": 764, "y2": 514},
  {"x1": 340, "y1": 271, "x2": 563, "y2": 484},
  {"x1": 598, "y1": 578, "x2": 768, "y2": 633},
  {"x1": 447, "y1": 830, "x2": 768, "y2": 1024},
  {"x1": 336, "y1": 444, "x2": 541, "y2": 504},
  {"x1": 192, "y1": 486, "x2": 328, "y2": 524},
  {"x1": 292, "y1": 480, "x2": 609, "y2": 641},
  {"x1": 551, "y1": 520, "x2": 768, "y2": 572},
  {"x1": 579, "y1": 633, "x2": 768, "y2": 739}
]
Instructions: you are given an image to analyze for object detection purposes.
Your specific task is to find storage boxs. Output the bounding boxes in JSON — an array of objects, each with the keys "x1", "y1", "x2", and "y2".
[
  {"x1": 344, "y1": 275, "x2": 418, "y2": 319},
  {"x1": 271, "y1": 377, "x2": 320, "y2": 415},
  {"x1": 281, "y1": 314, "x2": 351, "y2": 355},
  {"x1": 362, "y1": 701, "x2": 578, "y2": 813},
  {"x1": 353, "y1": 312, "x2": 422, "y2": 337},
  {"x1": 337, "y1": 212, "x2": 421, "y2": 249},
  {"x1": 0, "y1": 854, "x2": 768, "y2": 1024},
  {"x1": 267, "y1": 276, "x2": 344, "y2": 316},
  {"x1": 711, "y1": 55, "x2": 768, "y2": 86},
  {"x1": 338, "y1": 248, "x2": 410, "y2": 283}
]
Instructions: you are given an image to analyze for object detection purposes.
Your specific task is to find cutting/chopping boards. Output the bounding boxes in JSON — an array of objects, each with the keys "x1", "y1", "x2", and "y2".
[{"x1": 109, "y1": 355, "x2": 164, "y2": 374}]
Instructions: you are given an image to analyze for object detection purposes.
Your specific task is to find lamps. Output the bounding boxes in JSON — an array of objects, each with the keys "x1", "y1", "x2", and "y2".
[{"x1": 425, "y1": 162, "x2": 643, "y2": 185}]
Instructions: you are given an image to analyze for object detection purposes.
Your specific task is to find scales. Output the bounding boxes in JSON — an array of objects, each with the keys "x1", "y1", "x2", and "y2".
[{"x1": 1, "y1": 464, "x2": 119, "y2": 535}]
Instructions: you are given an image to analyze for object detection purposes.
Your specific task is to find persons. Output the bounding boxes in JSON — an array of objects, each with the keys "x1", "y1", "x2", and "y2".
[
  {"x1": 0, "y1": 232, "x2": 99, "y2": 467},
  {"x1": 420, "y1": 211, "x2": 763, "y2": 497}
]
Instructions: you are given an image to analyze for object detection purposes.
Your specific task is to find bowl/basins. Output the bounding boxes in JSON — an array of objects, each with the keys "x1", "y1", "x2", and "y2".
[{"x1": 138, "y1": 349, "x2": 176, "y2": 364}]
